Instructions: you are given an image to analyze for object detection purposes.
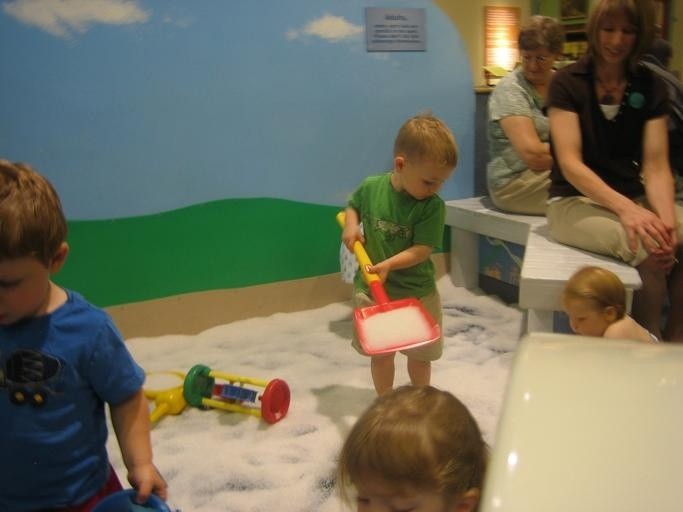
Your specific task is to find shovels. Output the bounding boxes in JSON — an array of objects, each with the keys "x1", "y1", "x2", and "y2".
[{"x1": 336, "y1": 212, "x2": 441, "y2": 356}]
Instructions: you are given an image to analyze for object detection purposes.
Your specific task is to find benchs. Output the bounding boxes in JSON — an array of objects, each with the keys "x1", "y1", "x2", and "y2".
[
  {"x1": 471, "y1": 330, "x2": 682, "y2": 512},
  {"x1": 438, "y1": 191, "x2": 644, "y2": 340}
]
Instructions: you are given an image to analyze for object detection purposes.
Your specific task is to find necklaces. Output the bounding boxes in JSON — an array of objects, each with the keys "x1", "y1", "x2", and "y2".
[{"x1": 594, "y1": 65, "x2": 626, "y2": 106}]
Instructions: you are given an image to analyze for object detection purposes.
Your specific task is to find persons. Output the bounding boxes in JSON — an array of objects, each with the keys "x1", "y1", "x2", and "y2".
[
  {"x1": 345, "y1": 114, "x2": 458, "y2": 395},
  {"x1": 3, "y1": 158, "x2": 170, "y2": 512},
  {"x1": 484, "y1": 15, "x2": 567, "y2": 216},
  {"x1": 335, "y1": 382, "x2": 488, "y2": 511},
  {"x1": 542, "y1": 0, "x2": 683, "y2": 341},
  {"x1": 559, "y1": 265, "x2": 658, "y2": 341}
]
